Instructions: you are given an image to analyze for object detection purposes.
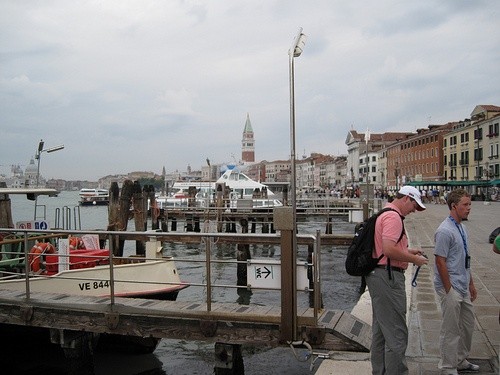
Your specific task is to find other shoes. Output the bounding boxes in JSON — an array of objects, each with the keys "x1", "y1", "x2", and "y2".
[{"x1": 457, "y1": 362, "x2": 480, "y2": 372}]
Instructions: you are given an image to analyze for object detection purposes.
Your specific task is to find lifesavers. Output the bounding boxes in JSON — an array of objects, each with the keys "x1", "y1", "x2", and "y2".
[
  {"x1": 201, "y1": 220, "x2": 212, "y2": 232},
  {"x1": 70, "y1": 237, "x2": 85, "y2": 250},
  {"x1": 28, "y1": 243, "x2": 44, "y2": 270},
  {"x1": 241, "y1": 219, "x2": 248, "y2": 226},
  {"x1": 32, "y1": 243, "x2": 58, "y2": 275}
]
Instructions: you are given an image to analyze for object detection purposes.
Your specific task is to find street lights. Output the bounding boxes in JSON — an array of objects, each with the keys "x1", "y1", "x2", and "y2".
[
  {"x1": 33, "y1": 138, "x2": 66, "y2": 220},
  {"x1": 288, "y1": 26, "x2": 308, "y2": 353},
  {"x1": 394, "y1": 161, "x2": 399, "y2": 192},
  {"x1": 364, "y1": 127, "x2": 371, "y2": 184},
  {"x1": 485, "y1": 161, "x2": 489, "y2": 202}
]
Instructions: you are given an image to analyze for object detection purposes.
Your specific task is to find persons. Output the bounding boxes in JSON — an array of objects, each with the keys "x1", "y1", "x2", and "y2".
[
  {"x1": 366, "y1": 185, "x2": 429, "y2": 375},
  {"x1": 374, "y1": 189, "x2": 397, "y2": 202},
  {"x1": 493, "y1": 234, "x2": 500, "y2": 253},
  {"x1": 428, "y1": 188, "x2": 448, "y2": 204},
  {"x1": 422, "y1": 189, "x2": 426, "y2": 196},
  {"x1": 330, "y1": 188, "x2": 360, "y2": 198},
  {"x1": 434, "y1": 189, "x2": 480, "y2": 375}
]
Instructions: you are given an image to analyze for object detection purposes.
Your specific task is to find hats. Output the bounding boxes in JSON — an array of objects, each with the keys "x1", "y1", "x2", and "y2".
[{"x1": 398, "y1": 186, "x2": 426, "y2": 211}]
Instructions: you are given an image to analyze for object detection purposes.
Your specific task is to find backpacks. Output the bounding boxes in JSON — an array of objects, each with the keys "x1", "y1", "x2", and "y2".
[{"x1": 345, "y1": 208, "x2": 405, "y2": 276}]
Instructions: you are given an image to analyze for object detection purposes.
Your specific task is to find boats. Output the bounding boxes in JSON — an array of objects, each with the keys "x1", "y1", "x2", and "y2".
[
  {"x1": 1, "y1": 179, "x2": 197, "y2": 358},
  {"x1": 78, "y1": 169, "x2": 284, "y2": 213}
]
